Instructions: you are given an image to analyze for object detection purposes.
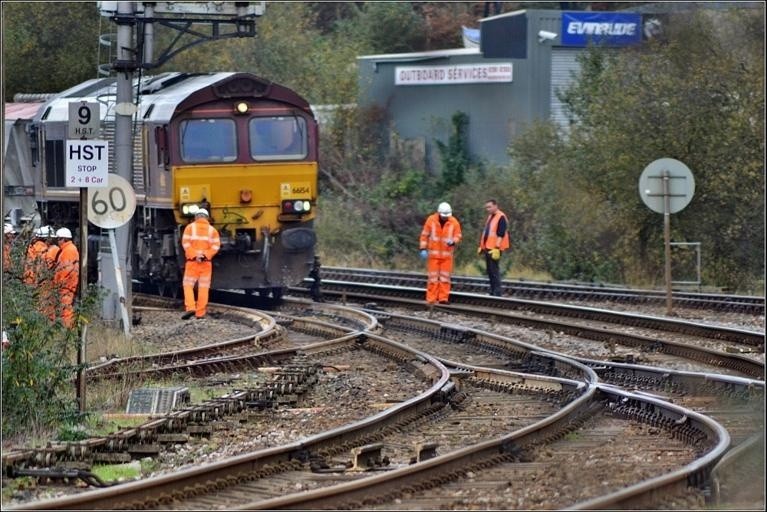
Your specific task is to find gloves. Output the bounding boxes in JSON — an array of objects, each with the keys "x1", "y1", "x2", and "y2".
[
  {"x1": 420, "y1": 249, "x2": 428, "y2": 261},
  {"x1": 477, "y1": 247, "x2": 482, "y2": 257},
  {"x1": 488, "y1": 248, "x2": 501, "y2": 260},
  {"x1": 447, "y1": 239, "x2": 455, "y2": 247}
]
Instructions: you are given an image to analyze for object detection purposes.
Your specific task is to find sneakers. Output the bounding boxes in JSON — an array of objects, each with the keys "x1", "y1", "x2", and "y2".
[
  {"x1": 181, "y1": 309, "x2": 195, "y2": 320},
  {"x1": 196, "y1": 316, "x2": 205, "y2": 319},
  {"x1": 439, "y1": 300, "x2": 450, "y2": 305}
]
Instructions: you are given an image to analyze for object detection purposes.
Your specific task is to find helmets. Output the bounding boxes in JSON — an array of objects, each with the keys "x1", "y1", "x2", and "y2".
[
  {"x1": 195, "y1": 208, "x2": 209, "y2": 219},
  {"x1": 55, "y1": 227, "x2": 73, "y2": 239},
  {"x1": 36, "y1": 225, "x2": 57, "y2": 238},
  {"x1": 438, "y1": 202, "x2": 453, "y2": 217},
  {"x1": 3, "y1": 222, "x2": 17, "y2": 235}
]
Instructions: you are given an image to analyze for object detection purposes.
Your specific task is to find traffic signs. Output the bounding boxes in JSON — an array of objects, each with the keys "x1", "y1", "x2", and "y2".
[{"x1": 87, "y1": 172, "x2": 137, "y2": 231}]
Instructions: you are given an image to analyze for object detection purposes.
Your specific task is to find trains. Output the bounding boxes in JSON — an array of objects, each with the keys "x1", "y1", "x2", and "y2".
[{"x1": 3, "y1": 65, "x2": 323, "y2": 309}]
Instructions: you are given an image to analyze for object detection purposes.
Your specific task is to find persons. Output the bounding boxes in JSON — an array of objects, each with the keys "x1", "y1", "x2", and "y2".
[
  {"x1": 1, "y1": 220, "x2": 79, "y2": 332},
  {"x1": 474, "y1": 199, "x2": 511, "y2": 296},
  {"x1": 181, "y1": 206, "x2": 221, "y2": 320},
  {"x1": 418, "y1": 199, "x2": 462, "y2": 306}
]
